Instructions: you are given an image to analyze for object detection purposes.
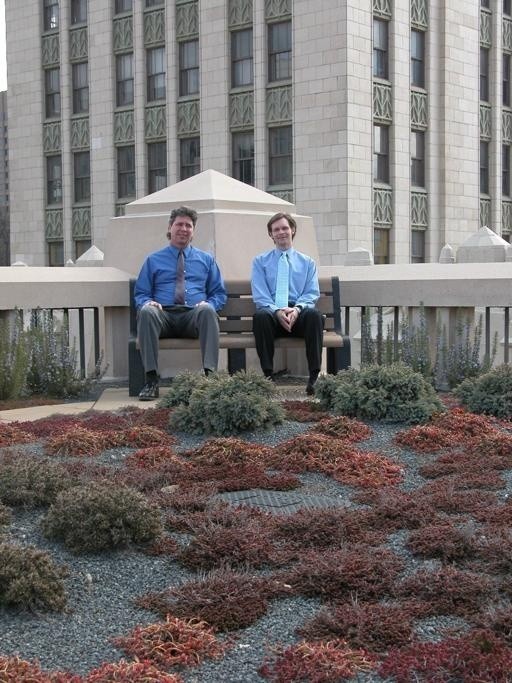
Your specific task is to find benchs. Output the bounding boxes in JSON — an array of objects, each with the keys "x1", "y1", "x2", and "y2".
[{"x1": 127, "y1": 276, "x2": 352, "y2": 395}]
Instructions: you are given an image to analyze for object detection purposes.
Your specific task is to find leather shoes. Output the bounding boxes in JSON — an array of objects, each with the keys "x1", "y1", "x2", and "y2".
[
  {"x1": 138, "y1": 382, "x2": 159, "y2": 400},
  {"x1": 307, "y1": 381, "x2": 316, "y2": 395}
]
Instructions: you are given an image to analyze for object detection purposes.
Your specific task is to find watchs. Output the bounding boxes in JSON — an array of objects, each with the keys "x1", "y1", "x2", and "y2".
[{"x1": 292, "y1": 306, "x2": 302, "y2": 315}]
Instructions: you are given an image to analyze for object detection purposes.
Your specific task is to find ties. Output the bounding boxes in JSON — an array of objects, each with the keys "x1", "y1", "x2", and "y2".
[
  {"x1": 275, "y1": 252, "x2": 289, "y2": 308},
  {"x1": 174, "y1": 250, "x2": 185, "y2": 304}
]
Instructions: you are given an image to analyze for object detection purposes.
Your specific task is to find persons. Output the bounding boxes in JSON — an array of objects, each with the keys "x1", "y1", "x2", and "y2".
[
  {"x1": 249, "y1": 213, "x2": 327, "y2": 396},
  {"x1": 133, "y1": 206, "x2": 228, "y2": 401}
]
